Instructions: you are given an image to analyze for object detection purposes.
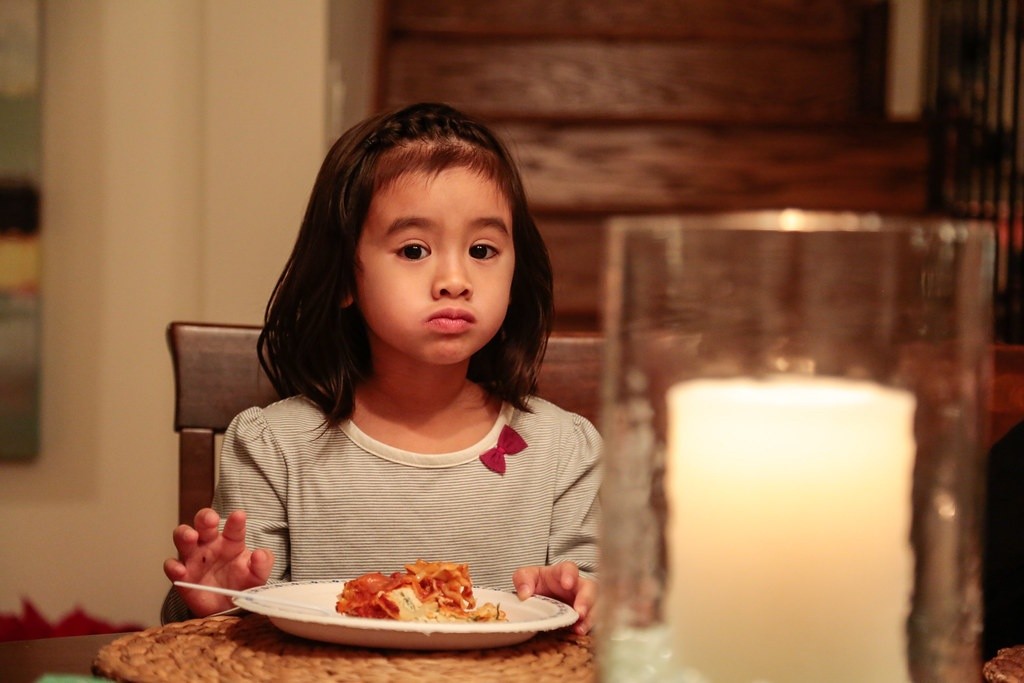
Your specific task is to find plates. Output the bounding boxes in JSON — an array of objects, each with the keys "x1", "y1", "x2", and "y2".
[{"x1": 232, "y1": 578, "x2": 578, "y2": 649}]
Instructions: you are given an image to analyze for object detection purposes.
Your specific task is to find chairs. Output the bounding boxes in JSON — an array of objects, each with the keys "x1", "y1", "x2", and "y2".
[{"x1": 167, "y1": 319, "x2": 1024, "y2": 566}]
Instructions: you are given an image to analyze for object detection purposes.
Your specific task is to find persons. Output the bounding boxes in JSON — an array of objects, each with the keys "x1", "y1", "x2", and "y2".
[{"x1": 160, "y1": 98, "x2": 604, "y2": 637}]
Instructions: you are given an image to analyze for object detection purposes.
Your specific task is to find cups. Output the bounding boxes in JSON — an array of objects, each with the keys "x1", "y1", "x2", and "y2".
[{"x1": 596, "y1": 218, "x2": 993, "y2": 683}]
